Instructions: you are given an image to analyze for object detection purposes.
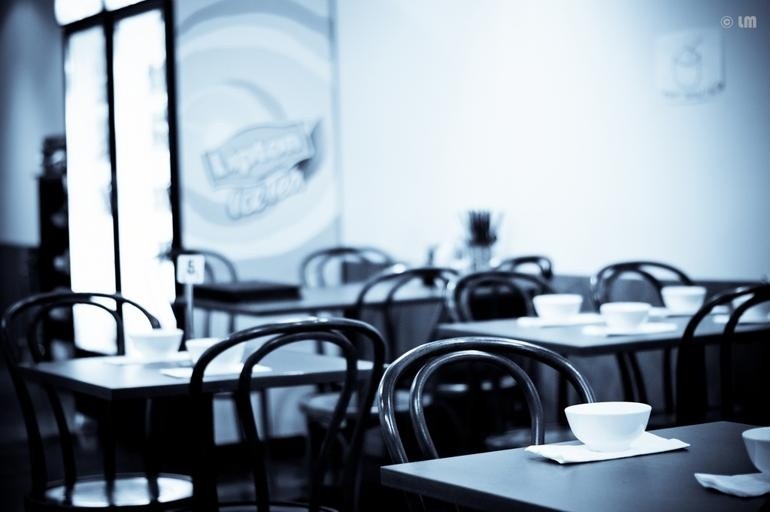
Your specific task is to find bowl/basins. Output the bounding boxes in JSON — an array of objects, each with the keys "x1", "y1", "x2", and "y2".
[
  {"x1": 563, "y1": 400, "x2": 653, "y2": 454},
  {"x1": 531, "y1": 284, "x2": 769, "y2": 332},
  {"x1": 127, "y1": 329, "x2": 245, "y2": 370},
  {"x1": 740, "y1": 425, "x2": 770, "y2": 479}
]
